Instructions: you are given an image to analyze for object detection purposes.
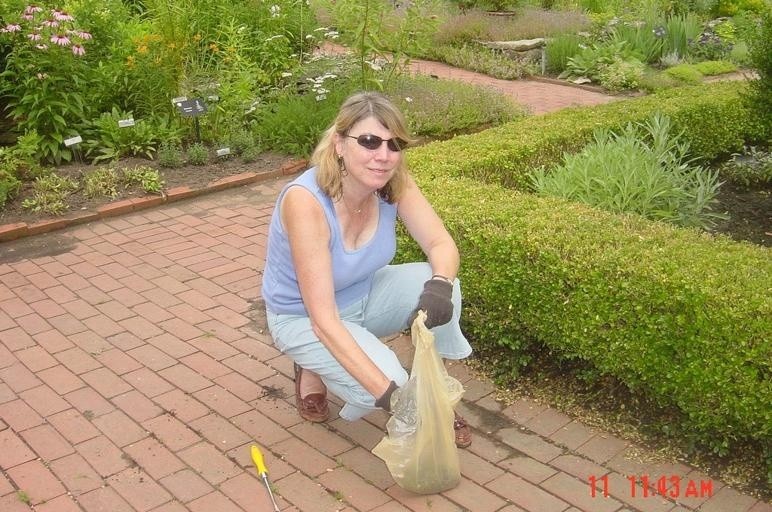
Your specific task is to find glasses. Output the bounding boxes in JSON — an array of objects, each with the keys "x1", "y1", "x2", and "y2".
[{"x1": 346, "y1": 134, "x2": 407, "y2": 151}]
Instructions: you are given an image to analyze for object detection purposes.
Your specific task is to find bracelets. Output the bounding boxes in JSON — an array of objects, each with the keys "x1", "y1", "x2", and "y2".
[{"x1": 430, "y1": 274, "x2": 456, "y2": 287}]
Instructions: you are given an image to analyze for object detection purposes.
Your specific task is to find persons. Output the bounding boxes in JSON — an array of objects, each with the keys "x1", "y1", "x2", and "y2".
[{"x1": 257, "y1": 90, "x2": 474, "y2": 450}]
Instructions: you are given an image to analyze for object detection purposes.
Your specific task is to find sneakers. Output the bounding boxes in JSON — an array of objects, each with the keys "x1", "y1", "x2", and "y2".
[
  {"x1": 455, "y1": 411, "x2": 472, "y2": 448},
  {"x1": 294, "y1": 361, "x2": 329, "y2": 422}
]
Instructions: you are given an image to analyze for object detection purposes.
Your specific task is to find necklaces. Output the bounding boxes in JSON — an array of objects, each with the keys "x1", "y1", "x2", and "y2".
[{"x1": 340, "y1": 186, "x2": 373, "y2": 214}]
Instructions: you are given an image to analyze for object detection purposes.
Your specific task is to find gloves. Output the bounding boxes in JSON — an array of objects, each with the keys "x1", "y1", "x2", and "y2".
[{"x1": 407, "y1": 280, "x2": 454, "y2": 329}]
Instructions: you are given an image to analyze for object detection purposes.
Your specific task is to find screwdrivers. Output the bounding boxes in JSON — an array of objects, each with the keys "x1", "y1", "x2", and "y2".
[{"x1": 252, "y1": 446, "x2": 281, "y2": 512}]
